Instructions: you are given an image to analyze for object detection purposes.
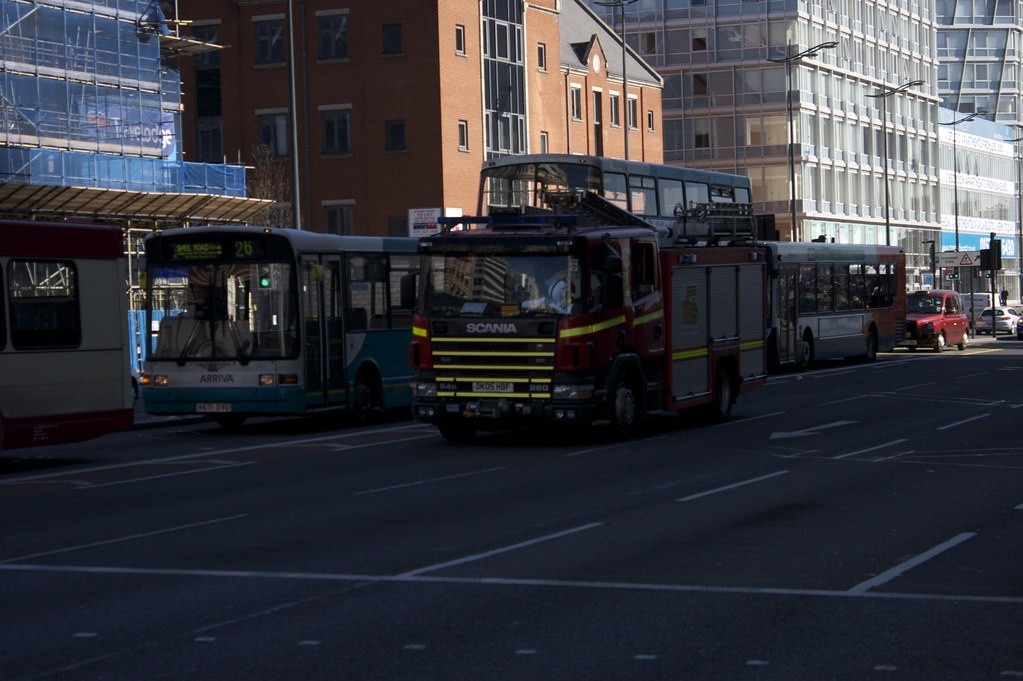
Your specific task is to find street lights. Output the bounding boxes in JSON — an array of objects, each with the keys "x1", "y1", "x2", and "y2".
[
  {"x1": 864, "y1": 79, "x2": 924, "y2": 294},
  {"x1": 765, "y1": 40, "x2": 837, "y2": 240},
  {"x1": 938, "y1": 111, "x2": 989, "y2": 252}
]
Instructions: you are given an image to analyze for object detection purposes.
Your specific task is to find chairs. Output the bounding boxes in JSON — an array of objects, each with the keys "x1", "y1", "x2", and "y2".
[{"x1": 304, "y1": 305, "x2": 414, "y2": 371}]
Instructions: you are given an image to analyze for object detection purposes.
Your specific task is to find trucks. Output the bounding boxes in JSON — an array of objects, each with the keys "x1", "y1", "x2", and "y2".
[{"x1": 960, "y1": 293, "x2": 1002, "y2": 328}]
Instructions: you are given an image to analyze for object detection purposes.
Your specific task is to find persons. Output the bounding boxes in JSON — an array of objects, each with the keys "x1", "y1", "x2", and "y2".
[
  {"x1": 1001, "y1": 287, "x2": 1008, "y2": 306},
  {"x1": 537, "y1": 263, "x2": 600, "y2": 309}
]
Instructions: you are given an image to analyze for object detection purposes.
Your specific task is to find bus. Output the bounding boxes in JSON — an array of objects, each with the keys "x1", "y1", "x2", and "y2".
[
  {"x1": 758, "y1": 242, "x2": 907, "y2": 365},
  {"x1": 0, "y1": 218, "x2": 137, "y2": 446},
  {"x1": 473, "y1": 153, "x2": 754, "y2": 243},
  {"x1": 141, "y1": 223, "x2": 421, "y2": 429}
]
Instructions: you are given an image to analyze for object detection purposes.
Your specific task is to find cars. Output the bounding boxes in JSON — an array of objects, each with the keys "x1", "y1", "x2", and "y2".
[
  {"x1": 896, "y1": 290, "x2": 968, "y2": 351},
  {"x1": 975, "y1": 306, "x2": 1019, "y2": 335},
  {"x1": 1017, "y1": 310, "x2": 1023, "y2": 340}
]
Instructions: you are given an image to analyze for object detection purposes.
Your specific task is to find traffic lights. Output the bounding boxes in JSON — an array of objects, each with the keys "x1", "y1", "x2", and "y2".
[
  {"x1": 948, "y1": 274, "x2": 959, "y2": 280},
  {"x1": 985, "y1": 274, "x2": 991, "y2": 278}
]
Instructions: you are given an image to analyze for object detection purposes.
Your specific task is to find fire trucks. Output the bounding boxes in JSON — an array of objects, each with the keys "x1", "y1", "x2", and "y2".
[{"x1": 410, "y1": 201, "x2": 771, "y2": 432}]
